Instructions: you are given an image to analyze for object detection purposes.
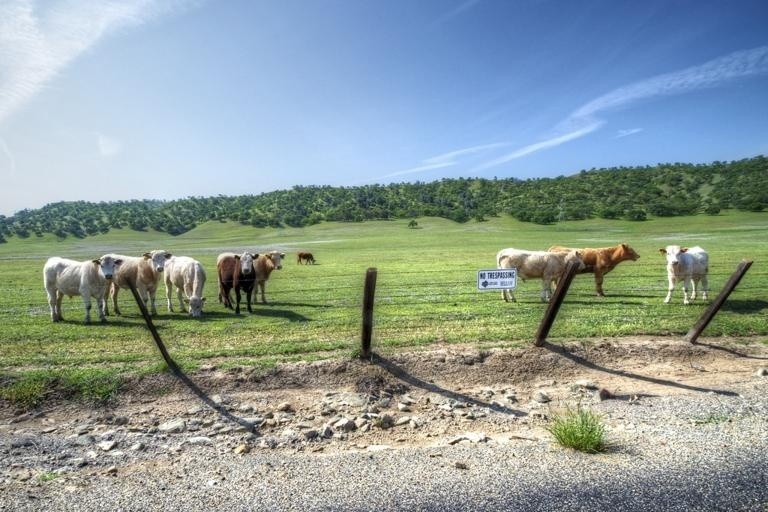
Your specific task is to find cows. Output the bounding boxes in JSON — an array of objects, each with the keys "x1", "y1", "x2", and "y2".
[
  {"x1": 496, "y1": 247, "x2": 587, "y2": 303},
  {"x1": 216, "y1": 250, "x2": 285, "y2": 316},
  {"x1": 98, "y1": 249, "x2": 171, "y2": 316},
  {"x1": 163, "y1": 254, "x2": 208, "y2": 318},
  {"x1": 548, "y1": 242, "x2": 641, "y2": 297},
  {"x1": 658, "y1": 245, "x2": 709, "y2": 305},
  {"x1": 296, "y1": 251, "x2": 317, "y2": 264},
  {"x1": 42, "y1": 254, "x2": 124, "y2": 325}
]
